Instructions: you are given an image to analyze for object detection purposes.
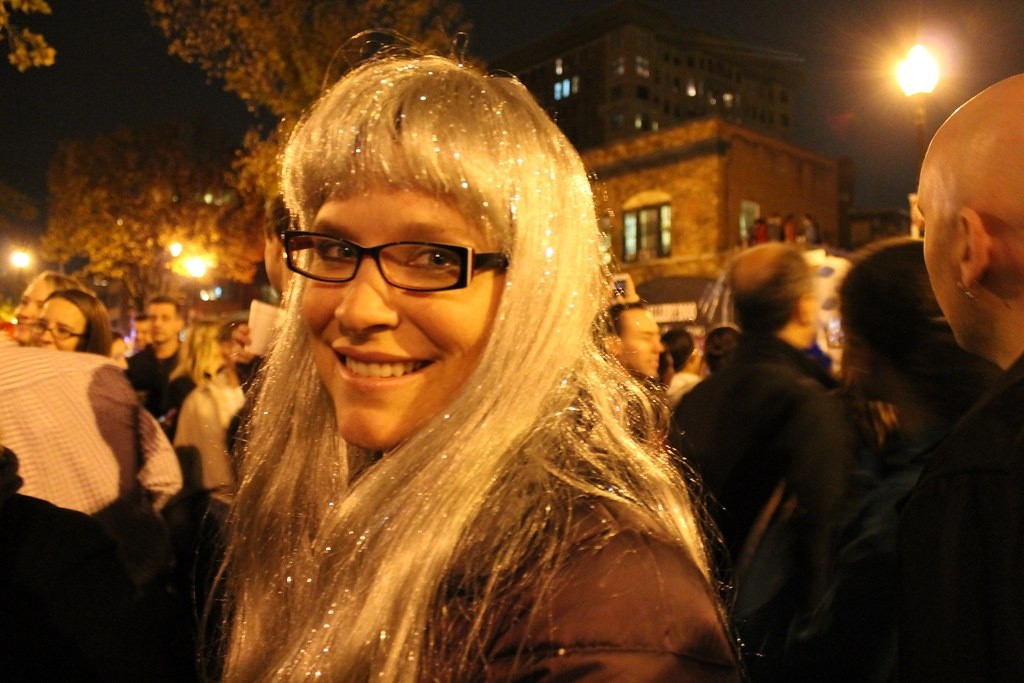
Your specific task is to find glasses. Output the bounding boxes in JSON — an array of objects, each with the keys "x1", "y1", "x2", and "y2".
[
  {"x1": 280, "y1": 231, "x2": 511, "y2": 293},
  {"x1": 31, "y1": 321, "x2": 85, "y2": 341}
]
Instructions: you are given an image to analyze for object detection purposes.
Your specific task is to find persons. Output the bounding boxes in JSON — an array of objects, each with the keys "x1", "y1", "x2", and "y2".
[{"x1": 0, "y1": 53, "x2": 1024, "y2": 683}]
